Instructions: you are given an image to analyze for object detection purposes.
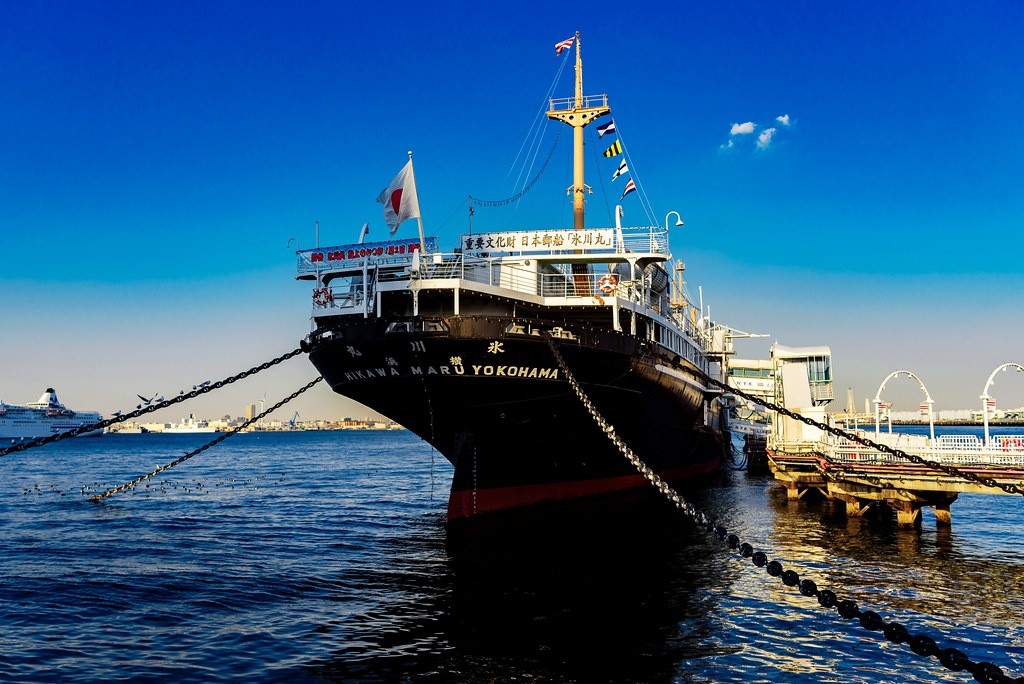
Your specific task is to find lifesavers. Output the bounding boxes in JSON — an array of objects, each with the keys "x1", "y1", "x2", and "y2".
[
  {"x1": 1003, "y1": 437, "x2": 1022, "y2": 453},
  {"x1": 312, "y1": 287, "x2": 330, "y2": 305},
  {"x1": 597, "y1": 274, "x2": 617, "y2": 293}
]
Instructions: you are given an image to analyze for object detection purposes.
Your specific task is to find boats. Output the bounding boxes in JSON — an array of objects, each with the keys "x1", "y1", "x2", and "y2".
[
  {"x1": 286, "y1": 30, "x2": 773, "y2": 527},
  {"x1": 0, "y1": 389, "x2": 106, "y2": 438}
]
